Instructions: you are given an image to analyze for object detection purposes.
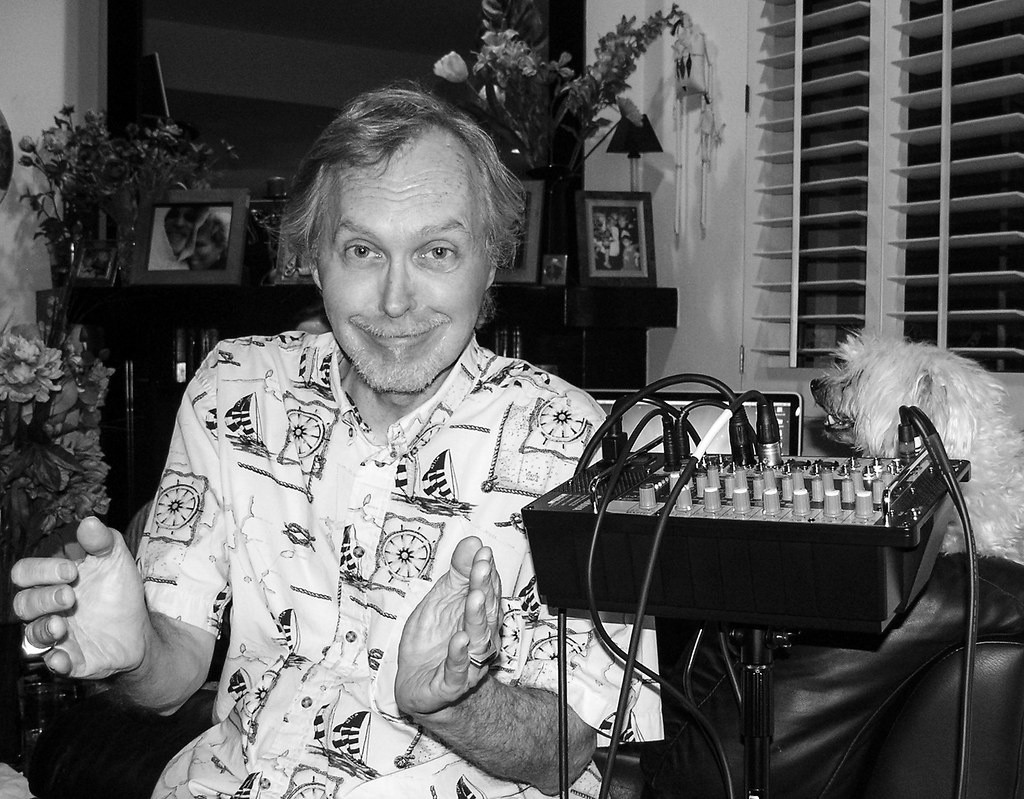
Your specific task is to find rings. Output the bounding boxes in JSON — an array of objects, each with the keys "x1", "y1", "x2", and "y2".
[
  {"x1": 465, "y1": 636, "x2": 500, "y2": 667},
  {"x1": 18, "y1": 636, "x2": 55, "y2": 656}
]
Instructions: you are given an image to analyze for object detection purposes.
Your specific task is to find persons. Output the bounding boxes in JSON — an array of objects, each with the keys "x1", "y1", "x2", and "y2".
[
  {"x1": 163, "y1": 206, "x2": 227, "y2": 269},
  {"x1": 0, "y1": 114, "x2": 53, "y2": 333},
  {"x1": 11, "y1": 86, "x2": 666, "y2": 798},
  {"x1": 594, "y1": 209, "x2": 641, "y2": 271}
]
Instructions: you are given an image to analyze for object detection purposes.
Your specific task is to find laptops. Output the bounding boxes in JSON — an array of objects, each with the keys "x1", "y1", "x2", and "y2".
[{"x1": 580, "y1": 389, "x2": 804, "y2": 459}]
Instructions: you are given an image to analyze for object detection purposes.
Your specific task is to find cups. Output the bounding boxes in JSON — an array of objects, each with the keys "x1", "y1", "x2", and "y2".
[{"x1": 16, "y1": 661, "x2": 79, "y2": 760}]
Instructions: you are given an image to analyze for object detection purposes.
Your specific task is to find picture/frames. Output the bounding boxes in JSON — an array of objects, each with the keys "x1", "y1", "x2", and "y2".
[
  {"x1": 578, "y1": 191, "x2": 656, "y2": 288},
  {"x1": 492, "y1": 173, "x2": 549, "y2": 286},
  {"x1": 71, "y1": 241, "x2": 119, "y2": 288},
  {"x1": 130, "y1": 187, "x2": 250, "y2": 284}
]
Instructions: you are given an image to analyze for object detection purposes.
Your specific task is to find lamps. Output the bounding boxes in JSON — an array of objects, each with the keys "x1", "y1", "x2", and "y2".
[{"x1": 606, "y1": 114, "x2": 664, "y2": 190}]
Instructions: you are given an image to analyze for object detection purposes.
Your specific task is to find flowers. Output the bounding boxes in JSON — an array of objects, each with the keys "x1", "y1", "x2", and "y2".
[
  {"x1": 0, "y1": 324, "x2": 114, "y2": 621},
  {"x1": 19, "y1": 104, "x2": 238, "y2": 262},
  {"x1": 434, "y1": 0, "x2": 682, "y2": 180}
]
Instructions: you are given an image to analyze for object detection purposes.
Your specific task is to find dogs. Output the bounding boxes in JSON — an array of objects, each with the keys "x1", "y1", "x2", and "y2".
[{"x1": 811, "y1": 332, "x2": 1023, "y2": 559}]
